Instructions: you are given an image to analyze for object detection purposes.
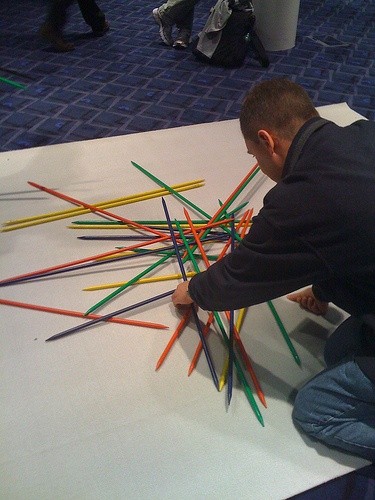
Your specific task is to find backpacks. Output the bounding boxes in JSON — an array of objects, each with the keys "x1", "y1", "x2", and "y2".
[{"x1": 192, "y1": 0, "x2": 269, "y2": 68}]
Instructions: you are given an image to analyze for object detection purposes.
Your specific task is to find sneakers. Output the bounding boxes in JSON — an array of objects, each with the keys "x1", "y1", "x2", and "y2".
[
  {"x1": 152, "y1": 8, "x2": 174, "y2": 45},
  {"x1": 173, "y1": 28, "x2": 190, "y2": 48}
]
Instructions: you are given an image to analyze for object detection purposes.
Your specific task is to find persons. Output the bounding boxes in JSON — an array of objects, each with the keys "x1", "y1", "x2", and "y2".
[
  {"x1": 172, "y1": 76, "x2": 375, "y2": 457},
  {"x1": 38, "y1": 0, "x2": 109, "y2": 49},
  {"x1": 152, "y1": 0, "x2": 195, "y2": 49}
]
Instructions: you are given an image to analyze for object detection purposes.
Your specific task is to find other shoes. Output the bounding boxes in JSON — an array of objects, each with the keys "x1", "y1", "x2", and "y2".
[
  {"x1": 37, "y1": 21, "x2": 74, "y2": 53},
  {"x1": 92, "y1": 19, "x2": 109, "y2": 37}
]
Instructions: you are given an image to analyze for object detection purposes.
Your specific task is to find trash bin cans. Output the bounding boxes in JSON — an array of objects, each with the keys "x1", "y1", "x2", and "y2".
[{"x1": 251, "y1": 0, "x2": 300, "y2": 52}]
